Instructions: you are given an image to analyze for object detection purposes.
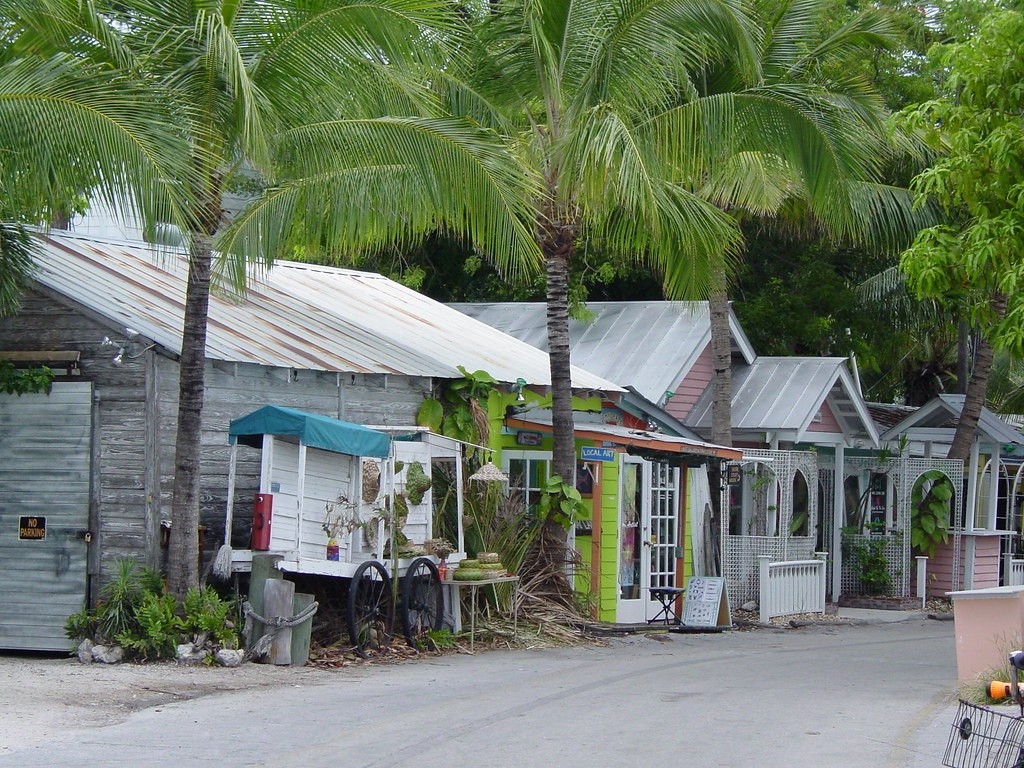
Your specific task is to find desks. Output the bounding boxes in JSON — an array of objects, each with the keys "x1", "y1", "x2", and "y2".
[{"x1": 441, "y1": 576, "x2": 520, "y2": 654}]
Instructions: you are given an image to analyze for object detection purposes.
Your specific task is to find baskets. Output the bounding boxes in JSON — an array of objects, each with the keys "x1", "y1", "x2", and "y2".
[
  {"x1": 365, "y1": 517, "x2": 380, "y2": 548},
  {"x1": 424, "y1": 539, "x2": 443, "y2": 554},
  {"x1": 942, "y1": 698, "x2": 1024, "y2": 768}
]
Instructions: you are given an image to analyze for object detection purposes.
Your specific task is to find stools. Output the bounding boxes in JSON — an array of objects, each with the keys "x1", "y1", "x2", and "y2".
[{"x1": 646, "y1": 585, "x2": 686, "y2": 627}]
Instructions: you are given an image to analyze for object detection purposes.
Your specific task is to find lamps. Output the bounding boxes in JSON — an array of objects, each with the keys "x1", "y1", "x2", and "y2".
[
  {"x1": 512, "y1": 379, "x2": 525, "y2": 400},
  {"x1": 661, "y1": 390, "x2": 674, "y2": 409},
  {"x1": 720, "y1": 464, "x2": 742, "y2": 486},
  {"x1": 99, "y1": 328, "x2": 139, "y2": 368}
]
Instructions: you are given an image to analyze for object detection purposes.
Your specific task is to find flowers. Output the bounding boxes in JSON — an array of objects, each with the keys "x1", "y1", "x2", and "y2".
[{"x1": 430, "y1": 537, "x2": 454, "y2": 560}]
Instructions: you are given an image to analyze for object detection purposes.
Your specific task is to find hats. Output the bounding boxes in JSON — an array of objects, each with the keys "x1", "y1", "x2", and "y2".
[
  {"x1": 362, "y1": 460, "x2": 381, "y2": 502},
  {"x1": 406, "y1": 460, "x2": 431, "y2": 505},
  {"x1": 385, "y1": 494, "x2": 409, "y2": 516}
]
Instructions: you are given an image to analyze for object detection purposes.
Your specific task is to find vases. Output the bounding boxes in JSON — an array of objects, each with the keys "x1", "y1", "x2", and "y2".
[
  {"x1": 327, "y1": 537, "x2": 339, "y2": 560},
  {"x1": 438, "y1": 559, "x2": 447, "y2": 581}
]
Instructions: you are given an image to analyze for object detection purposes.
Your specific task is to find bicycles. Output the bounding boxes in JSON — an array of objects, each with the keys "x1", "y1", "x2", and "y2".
[{"x1": 942, "y1": 651, "x2": 1024, "y2": 768}]
